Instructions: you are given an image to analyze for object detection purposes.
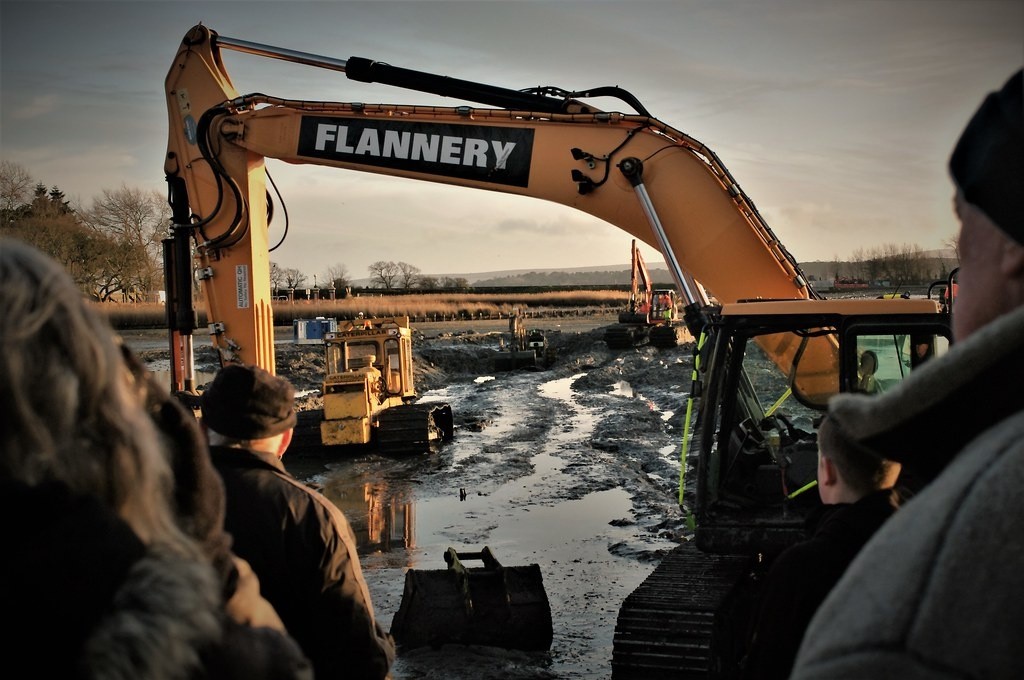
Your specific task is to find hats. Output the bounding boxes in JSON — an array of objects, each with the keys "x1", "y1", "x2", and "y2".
[
  {"x1": 947, "y1": 67, "x2": 1024, "y2": 247},
  {"x1": 203, "y1": 364, "x2": 297, "y2": 437}
]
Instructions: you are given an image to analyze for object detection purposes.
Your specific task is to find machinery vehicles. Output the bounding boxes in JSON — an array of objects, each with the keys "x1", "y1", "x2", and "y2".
[
  {"x1": 491, "y1": 302, "x2": 553, "y2": 372},
  {"x1": 162, "y1": 20, "x2": 960, "y2": 680},
  {"x1": 605, "y1": 238, "x2": 695, "y2": 350},
  {"x1": 320, "y1": 311, "x2": 455, "y2": 455}
]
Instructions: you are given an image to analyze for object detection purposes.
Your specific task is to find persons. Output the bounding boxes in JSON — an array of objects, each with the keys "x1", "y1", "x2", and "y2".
[
  {"x1": 662, "y1": 291, "x2": 672, "y2": 327},
  {"x1": 744, "y1": 392, "x2": 905, "y2": 679},
  {"x1": 203, "y1": 364, "x2": 393, "y2": 680},
  {"x1": 0, "y1": 241, "x2": 309, "y2": 680},
  {"x1": 790, "y1": 67, "x2": 1023, "y2": 679}
]
